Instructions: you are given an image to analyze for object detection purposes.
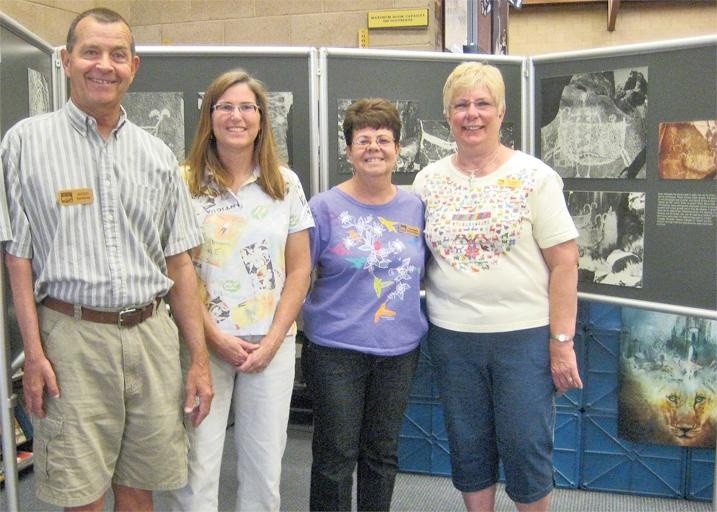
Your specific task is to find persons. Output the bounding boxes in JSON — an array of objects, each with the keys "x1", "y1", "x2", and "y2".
[
  {"x1": 172, "y1": 70, "x2": 314, "y2": 510},
  {"x1": 0, "y1": 7, "x2": 214, "y2": 512},
  {"x1": 404, "y1": 60, "x2": 588, "y2": 511},
  {"x1": 296, "y1": 97, "x2": 428, "y2": 511}
]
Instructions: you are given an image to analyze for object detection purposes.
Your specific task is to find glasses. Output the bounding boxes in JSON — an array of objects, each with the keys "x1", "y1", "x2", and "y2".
[
  {"x1": 211, "y1": 103, "x2": 260, "y2": 114},
  {"x1": 356, "y1": 134, "x2": 396, "y2": 146}
]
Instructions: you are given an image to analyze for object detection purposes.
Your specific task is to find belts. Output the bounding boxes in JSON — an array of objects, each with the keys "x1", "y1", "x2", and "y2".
[{"x1": 40, "y1": 295, "x2": 161, "y2": 329}]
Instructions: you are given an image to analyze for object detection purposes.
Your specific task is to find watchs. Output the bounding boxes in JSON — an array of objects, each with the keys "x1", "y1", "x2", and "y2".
[{"x1": 548, "y1": 331, "x2": 575, "y2": 344}]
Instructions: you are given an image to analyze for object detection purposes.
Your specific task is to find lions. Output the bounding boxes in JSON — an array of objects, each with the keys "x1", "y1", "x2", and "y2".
[{"x1": 647, "y1": 360, "x2": 716, "y2": 447}]
